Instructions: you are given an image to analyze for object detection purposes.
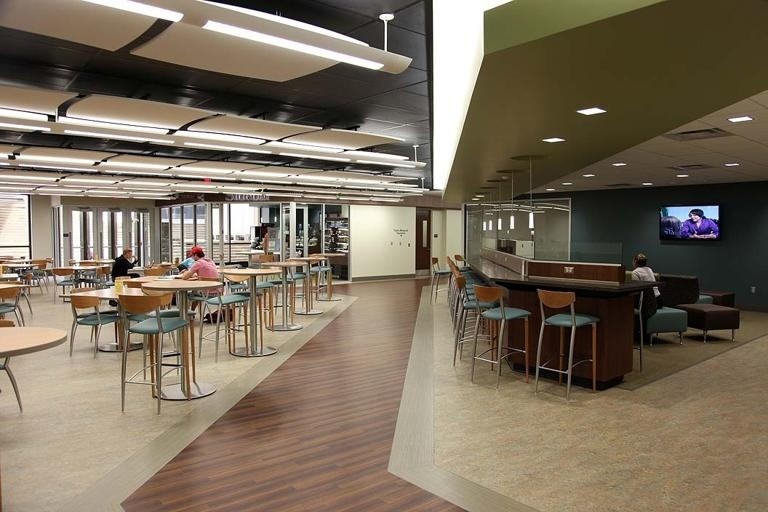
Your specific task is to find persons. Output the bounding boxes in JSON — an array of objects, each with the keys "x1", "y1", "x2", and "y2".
[
  {"x1": 177, "y1": 251, "x2": 195, "y2": 270},
  {"x1": 680, "y1": 208, "x2": 718, "y2": 238},
  {"x1": 182, "y1": 245, "x2": 219, "y2": 311},
  {"x1": 110, "y1": 248, "x2": 140, "y2": 281},
  {"x1": 631, "y1": 253, "x2": 663, "y2": 343}
]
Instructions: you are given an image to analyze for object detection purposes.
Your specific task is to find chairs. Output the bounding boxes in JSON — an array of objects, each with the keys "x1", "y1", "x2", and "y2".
[
  {"x1": 470, "y1": 280, "x2": 531, "y2": 387},
  {"x1": 532, "y1": 287, "x2": 600, "y2": 399},
  {"x1": 0, "y1": 252, "x2": 335, "y2": 420},
  {"x1": 423, "y1": 248, "x2": 499, "y2": 373}
]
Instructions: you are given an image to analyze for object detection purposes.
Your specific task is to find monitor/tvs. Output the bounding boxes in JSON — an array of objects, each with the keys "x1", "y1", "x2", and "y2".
[{"x1": 656, "y1": 203, "x2": 722, "y2": 244}]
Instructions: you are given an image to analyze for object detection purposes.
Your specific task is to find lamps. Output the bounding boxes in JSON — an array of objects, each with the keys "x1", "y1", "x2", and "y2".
[
  {"x1": 0, "y1": 106, "x2": 440, "y2": 204},
  {"x1": 85, "y1": 1, "x2": 417, "y2": 77}
]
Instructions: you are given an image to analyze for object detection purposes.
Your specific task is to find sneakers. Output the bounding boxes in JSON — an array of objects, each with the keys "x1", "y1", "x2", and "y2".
[{"x1": 187, "y1": 310, "x2": 196, "y2": 315}]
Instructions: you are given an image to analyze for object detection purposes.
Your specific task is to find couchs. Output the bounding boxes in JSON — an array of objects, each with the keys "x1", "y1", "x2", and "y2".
[
  {"x1": 701, "y1": 289, "x2": 736, "y2": 307},
  {"x1": 624, "y1": 269, "x2": 660, "y2": 285},
  {"x1": 638, "y1": 305, "x2": 690, "y2": 349},
  {"x1": 660, "y1": 273, "x2": 713, "y2": 307},
  {"x1": 674, "y1": 303, "x2": 740, "y2": 346}
]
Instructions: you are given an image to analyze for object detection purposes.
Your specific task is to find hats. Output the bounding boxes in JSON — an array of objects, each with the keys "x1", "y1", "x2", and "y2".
[{"x1": 188, "y1": 247, "x2": 202, "y2": 257}]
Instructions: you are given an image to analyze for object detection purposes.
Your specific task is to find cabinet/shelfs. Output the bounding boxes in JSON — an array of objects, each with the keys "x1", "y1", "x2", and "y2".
[{"x1": 325, "y1": 218, "x2": 349, "y2": 254}]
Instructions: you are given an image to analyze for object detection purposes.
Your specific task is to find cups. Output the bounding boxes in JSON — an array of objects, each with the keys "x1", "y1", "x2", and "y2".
[
  {"x1": 130, "y1": 253, "x2": 187, "y2": 278},
  {"x1": 115, "y1": 277, "x2": 125, "y2": 293}
]
